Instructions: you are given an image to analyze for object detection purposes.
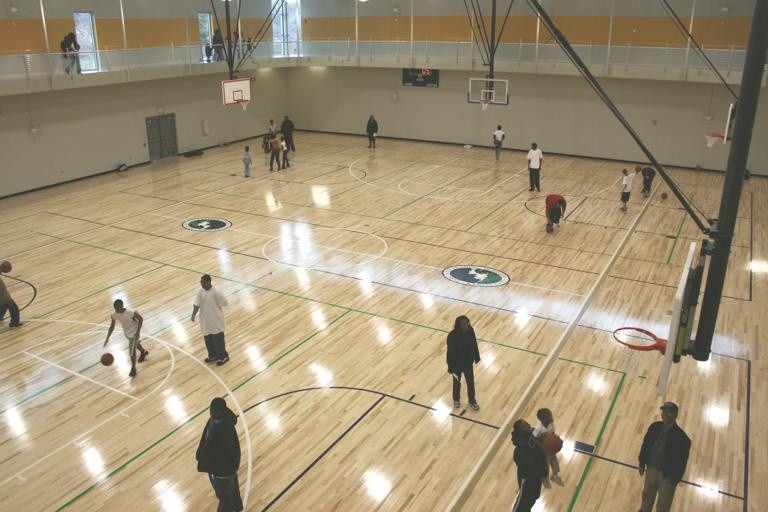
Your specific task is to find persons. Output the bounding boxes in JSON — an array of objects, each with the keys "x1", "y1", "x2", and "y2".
[
  {"x1": 447, "y1": 316, "x2": 479, "y2": 414},
  {"x1": 242, "y1": 147, "x2": 252, "y2": 177},
  {"x1": 282, "y1": 115, "x2": 295, "y2": 152},
  {"x1": 195, "y1": 397, "x2": 245, "y2": 512},
  {"x1": 493, "y1": 126, "x2": 504, "y2": 162},
  {"x1": 103, "y1": 299, "x2": 149, "y2": 376},
  {"x1": 280, "y1": 136, "x2": 291, "y2": 169},
  {"x1": 237, "y1": 33, "x2": 245, "y2": 59},
  {"x1": 247, "y1": 37, "x2": 255, "y2": 58},
  {"x1": 269, "y1": 120, "x2": 277, "y2": 139},
  {"x1": 0, "y1": 269, "x2": 22, "y2": 329},
  {"x1": 191, "y1": 275, "x2": 229, "y2": 366},
  {"x1": 545, "y1": 193, "x2": 567, "y2": 227},
  {"x1": 62, "y1": 36, "x2": 77, "y2": 58},
  {"x1": 640, "y1": 167, "x2": 657, "y2": 196},
  {"x1": 212, "y1": 29, "x2": 224, "y2": 61},
  {"x1": 533, "y1": 411, "x2": 565, "y2": 488},
  {"x1": 262, "y1": 136, "x2": 271, "y2": 153},
  {"x1": 205, "y1": 43, "x2": 213, "y2": 62},
  {"x1": 525, "y1": 142, "x2": 543, "y2": 192},
  {"x1": 509, "y1": 419, "x2": 549, "y2": 512},
  {"x1": 269, "y1": 136, "x2": 282, "y2": 171},
  {"x1": 619, "y1": 169, "x2": 637, "y2": 211},
  {"x1": 366, "y1": 116, "x2": 378, "y2": 149},
  {"x1": 60, "y1": 33, "x2": 81, "y2": 75},
  {"x1": 638, "y1": 402, "x2": 691, "y2": 511}
]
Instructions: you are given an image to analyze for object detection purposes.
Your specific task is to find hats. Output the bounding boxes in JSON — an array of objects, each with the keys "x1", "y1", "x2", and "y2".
[{"x1": 659, "y1": 401, "x2": 675, "y2": 411}]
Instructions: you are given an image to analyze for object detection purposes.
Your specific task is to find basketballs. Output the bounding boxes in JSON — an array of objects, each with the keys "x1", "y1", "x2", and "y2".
[
  {"x1": 2, "y1": 261, "x2": 12, "y2": 273},
  {"x1": 101, "y1": 353, "x2": 113, "y2": 366},
  {"x1": 635, "y1": 165, "x2": 641, "y2": 172},
  {"x1": 542, "y1": 434, "x2": 563, "y2": 454},
  {"x1": 546, "y1": 225, "x2": 553, "y2": 233},
  {"x1": 661, "y1": 193, "x2": 667, "y2": 199}
]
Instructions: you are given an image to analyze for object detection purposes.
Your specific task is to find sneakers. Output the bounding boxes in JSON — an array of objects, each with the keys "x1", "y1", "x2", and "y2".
[
  {"x1": 528, "y1": 187, "x2": 533, "y2": 191},
  {"x1": 8, "y1": 321, "x2": 22, "y2": 329},
  {"x1": 467, "y1": 397, "x2": 481, "y2": 415},
  {"x1": 213, "y1": 352, "x2": 231, "y2": 366},
  {"x1": 127, "y1": 365, "x2": 137, "y2": 376},
  {"x1": 452, "y1": 398, "x2": 457, "y2": 410},
  {"x1": 550, "y1": 475, "x2": 567, "y2": 488},
  {"x1": 135, "y1": 348, "x2": 151, "y2": 360},
  {"x1": 619, "y1": 206, "x2": 627, "y2": 212},
  {"x1": 367, "y1": 145, "x2": 372, "y2": 148},
  {"x1": 537, "y1": 472, "x2": 551, "y2": 490},
  {"x1": 203, "y1": 351, "x2": 213, "y2": 364}
]
